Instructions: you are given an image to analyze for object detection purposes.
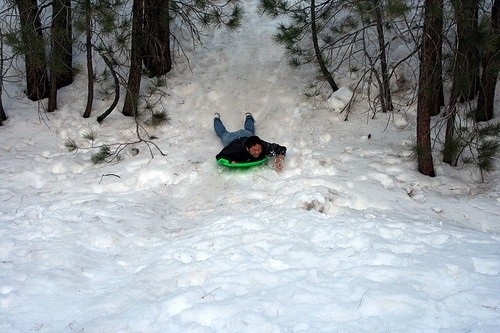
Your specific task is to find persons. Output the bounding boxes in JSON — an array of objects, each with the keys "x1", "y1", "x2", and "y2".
[{"x1": 212, "y1": 111, "x2": 288, "y2": 174}]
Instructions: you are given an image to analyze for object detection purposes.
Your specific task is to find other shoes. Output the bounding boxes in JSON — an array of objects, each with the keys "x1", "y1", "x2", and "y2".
[
  {"x1": 246, "y1": 113, "x2": 251, "y2": 116},
  {"x1": 213, "y1": 113, "x2": 219, "y2": 118}
]
